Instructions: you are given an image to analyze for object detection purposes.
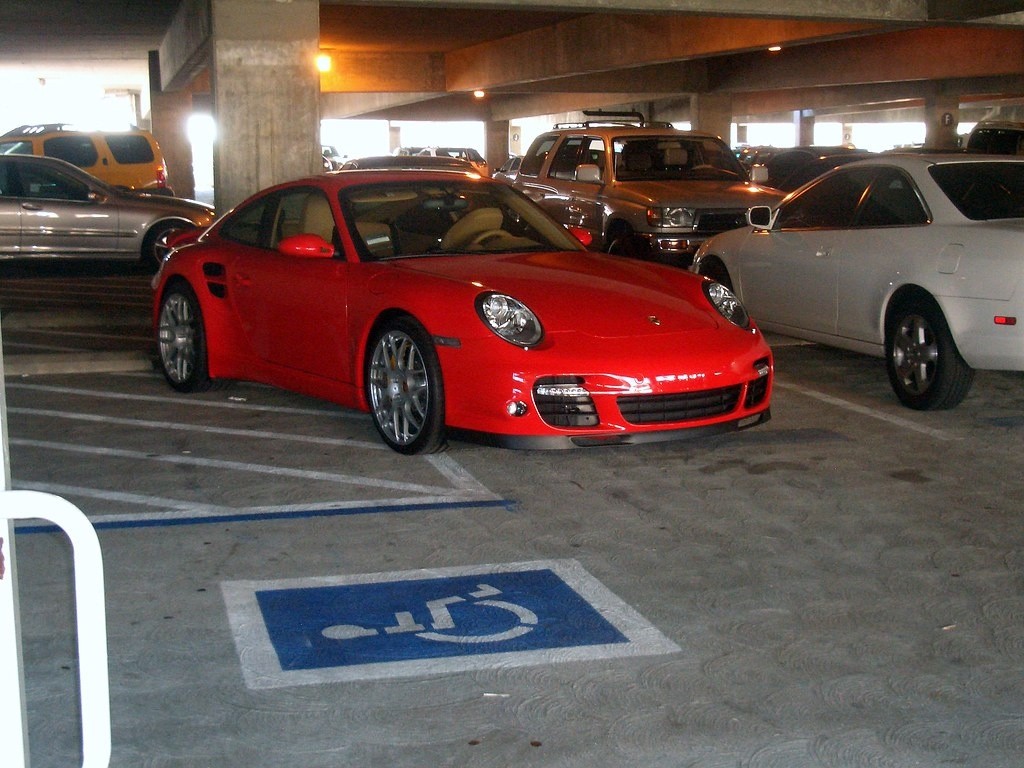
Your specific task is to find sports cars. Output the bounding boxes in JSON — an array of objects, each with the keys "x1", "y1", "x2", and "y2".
[
  {"x1": 151, "y1": 168, "x2": 777, "y2": 457},
  {"x1": 685, "y1": 151, "x2": 1024, "y2": 413}
]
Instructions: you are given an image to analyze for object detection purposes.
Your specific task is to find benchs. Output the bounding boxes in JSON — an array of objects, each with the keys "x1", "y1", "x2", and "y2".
[{"x1": 229, "y1": 220, "x2": 393, "y2": 256}]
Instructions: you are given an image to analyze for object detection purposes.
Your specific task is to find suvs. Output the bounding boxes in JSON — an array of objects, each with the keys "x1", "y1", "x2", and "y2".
[{"x1": 511, "y1": 122, "x2": 789, "y2": 268}]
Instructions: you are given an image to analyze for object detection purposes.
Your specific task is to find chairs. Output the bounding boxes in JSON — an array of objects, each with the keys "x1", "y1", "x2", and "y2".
[
  {"x1": 440, "y1": 207, "x2": 503, "y2": 250},
  {"x1": 539, "y1": 145, "x2": 693, "y2": 170},
  {"x1": 299, "y1": 195, "x2": 335, "y2": 242}
]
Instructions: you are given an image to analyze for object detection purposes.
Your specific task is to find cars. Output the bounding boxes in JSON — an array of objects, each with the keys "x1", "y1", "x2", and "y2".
[
  {"x1": 320, "y1": 118, "x2": 1024, "y2": 197},
  {"x1": 0, "y1": 152, "x2": 215, "y2": 273}
]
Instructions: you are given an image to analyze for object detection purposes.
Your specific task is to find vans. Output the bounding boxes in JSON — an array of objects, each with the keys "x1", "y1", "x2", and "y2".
[{"x1": 0, "y1": 121, "x2": 173, "y2": 196}]
